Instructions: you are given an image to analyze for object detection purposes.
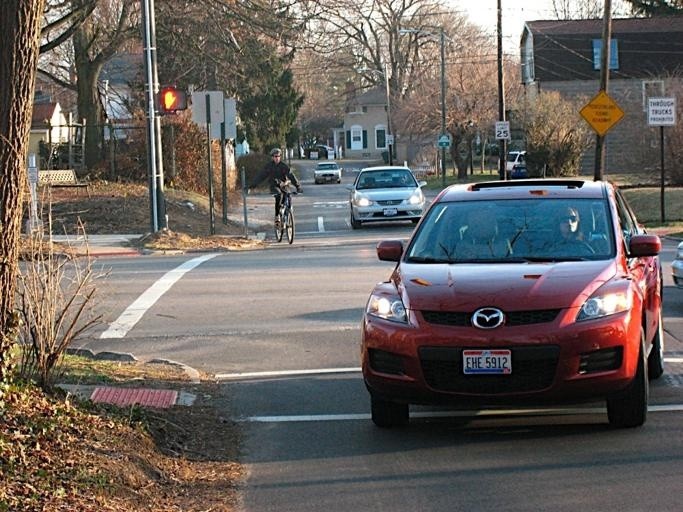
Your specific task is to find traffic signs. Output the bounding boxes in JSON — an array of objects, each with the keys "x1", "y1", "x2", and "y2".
[{"x1": 495, "y1": 120, "x2": 511, "y2": 141}]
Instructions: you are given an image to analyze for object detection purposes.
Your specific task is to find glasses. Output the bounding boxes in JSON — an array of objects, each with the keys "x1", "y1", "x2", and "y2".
[{"x1": 560, "y1": 216, "x2": 576, "y2": 223}]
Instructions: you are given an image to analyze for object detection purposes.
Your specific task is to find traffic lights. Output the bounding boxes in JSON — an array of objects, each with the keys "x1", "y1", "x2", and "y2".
[{"x1": 159, "y1": 88, "x2": 188, "y2": 111}]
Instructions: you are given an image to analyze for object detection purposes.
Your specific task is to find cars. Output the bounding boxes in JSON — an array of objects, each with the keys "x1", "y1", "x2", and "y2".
[
  {"x1": 360, "y1": 177, "x2": 665, "y2": 429},
  {"x1": 345, "y1": 166, "x2": 428, "y2": 229},
  {"x1": 314, "y1": 144, "x2": 333, "y2": 150},
  {"x1": 313, "y1": 162, "x2": 343, "y2": 184}
]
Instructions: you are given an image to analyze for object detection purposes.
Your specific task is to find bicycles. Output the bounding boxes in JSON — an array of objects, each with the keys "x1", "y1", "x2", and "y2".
[{"x1": 274, "y1": 185, "x2": 304, "y2": 244}]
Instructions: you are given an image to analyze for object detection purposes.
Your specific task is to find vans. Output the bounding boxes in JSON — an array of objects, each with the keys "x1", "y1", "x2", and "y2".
[{"x1": 497, "y1": 150, "x2": 552, "y2": 180}]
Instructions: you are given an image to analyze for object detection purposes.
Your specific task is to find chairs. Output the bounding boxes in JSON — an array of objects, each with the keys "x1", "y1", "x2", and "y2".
[
  {"x1": 362, "y1": 176, "x2": 409, "y2": 187},
  {"x1": 451, "y1": 208, "x2": 606, "y2": 258}
]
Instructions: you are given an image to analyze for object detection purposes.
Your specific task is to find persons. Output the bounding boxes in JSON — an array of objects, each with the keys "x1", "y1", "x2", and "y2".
[
  {"x1": 536, "y1": 205, "x2": 600, "y2": 256},
  {"x1": 249, "y1": 148, "x2": 304, "y2": 227}
]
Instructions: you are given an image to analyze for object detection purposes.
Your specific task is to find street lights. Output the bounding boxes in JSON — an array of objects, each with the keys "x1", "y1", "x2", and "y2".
[
  {"x1": 397, "y1": 26, "x2": 446, "y2": 190},
  {"x1": 356, "y1": 61, "x2": 393, "y2": 166}
]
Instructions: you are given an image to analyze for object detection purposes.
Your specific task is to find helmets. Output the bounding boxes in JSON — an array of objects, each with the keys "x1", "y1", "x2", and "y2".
[{"x1": 271, "y1": 148, "x2": 281, "y2": 155}]
